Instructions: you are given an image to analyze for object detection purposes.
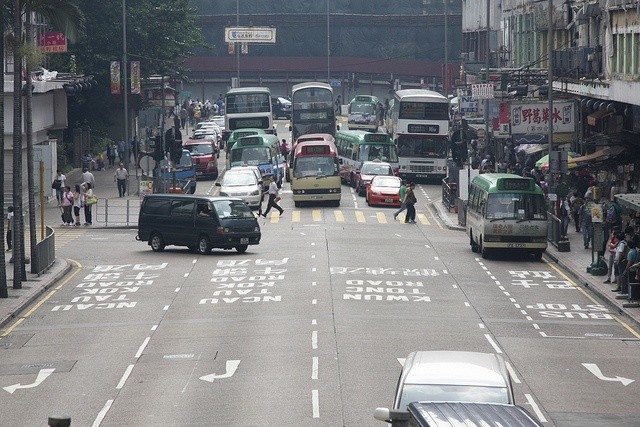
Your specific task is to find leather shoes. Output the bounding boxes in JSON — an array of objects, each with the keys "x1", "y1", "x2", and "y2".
[
  {"x1": 280, "y1": 210, "x2": 284, "y2": 215},
  {"x1": 261, "y1": 214, "x2": 266, "y2": 217}
]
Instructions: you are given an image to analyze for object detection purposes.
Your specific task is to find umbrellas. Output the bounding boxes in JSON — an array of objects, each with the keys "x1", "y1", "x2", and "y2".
[{"x1": 535, "y1": 147, "x2": 590, "y2": 172}]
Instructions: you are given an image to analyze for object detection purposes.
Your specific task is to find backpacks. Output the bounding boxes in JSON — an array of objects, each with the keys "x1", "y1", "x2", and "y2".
[{"x1": 621, "y1": 242, "x2": 630, "y2": 259}]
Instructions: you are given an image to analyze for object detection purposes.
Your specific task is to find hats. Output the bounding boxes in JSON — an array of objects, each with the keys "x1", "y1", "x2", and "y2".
[{"x1": 194, "y1": 106, "x2": 201, "y2": 110}]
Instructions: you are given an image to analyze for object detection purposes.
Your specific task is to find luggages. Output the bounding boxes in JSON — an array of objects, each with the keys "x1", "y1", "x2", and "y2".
[
  {"x1": 627, "y1": 266, "x2": 639, "y2": 303},
  {"x1": 59, "y1": 205, "x2": 74, "y2": 224}
]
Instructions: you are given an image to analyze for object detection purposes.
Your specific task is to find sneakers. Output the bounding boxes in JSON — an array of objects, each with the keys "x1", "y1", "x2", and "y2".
[
  {"x1": 74, "y1": 223, "x2": 78, "y2": 227},
  {"x1": 7, "y1": 247, "x2": 12, "y2": 251},
  {"x1": 78, "y1": 222, "x2": 81, "y2": 226},
  {"x1": 411, "y1": 221, "x2": 415, "y2": 224},
  {"x1": 64, "y1": 222, "x2": 67, "y2": 226},
  {"x1": 603, "y1": 279, "x2": 611, "y2": 284},
  {"x1": 84, "y1": 223, "x2": 90, "y2": 225},
  {"x1": 405, "y1": 222, "x2": 411, "y2": 223},
  {"x1": 69, "y1": 222, "x2": 72, "y2": 226},
  {"x1": 612, "y1": 281, "x2": 617, "y2": 284},
  {"x1": 393, "y1": 213, "x2": 396, "y2": 220},
  {"x1": 90, "y1": 222, "x2": 92, "y2": 225},
  {"x1": 414, "y1": 222, "x2": 417, "y2": 223}
]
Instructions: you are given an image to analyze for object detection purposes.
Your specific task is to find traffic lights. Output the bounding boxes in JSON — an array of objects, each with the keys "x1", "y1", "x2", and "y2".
[
  {"x1": 171, "y1": 137, "x2": 182, "y2": 158},
  {"x1": 145, "y1": 134, "x2": 160, "y2": 160}
]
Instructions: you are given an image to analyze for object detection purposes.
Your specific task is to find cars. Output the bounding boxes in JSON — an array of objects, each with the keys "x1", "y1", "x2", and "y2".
[
  {"x1": 189, "y1": 129, "x2": 221, "y2": 158},
  {"x1": 199, "y1": 125, "x2": 222, "y2": 142},
  {"x1": 230, "y1": 163, "x2": 265, "y2": 200},
  {"x1": 192, "y1": 121, "x2": 222, "y2": 136},
  {"x1": 355, "y1": 158, "x2": 395, "y2": 195},
  {"x1": 213, "y1": 113, "x2": 225, "y2": 118},
  {"x1": 209, "y1": 118, "x2": 226, "y2": 137},
  {"x1": 373, "y1": 349, "x2": 515, "y2": 426},
  {"x1": 366, "y1": 174, "x2": 408, "y2": 206},
  {"x1": 215, "y1": 169, "x2": 263, "y2": 208}
]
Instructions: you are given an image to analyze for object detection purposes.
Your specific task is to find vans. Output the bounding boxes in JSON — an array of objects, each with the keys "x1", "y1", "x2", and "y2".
[
  {"x1": 182, "y1": 139, "x2": 219, "y2": 179},
  {"x1": 135, "y1": 192, "x2": 261, "y2": 254}
]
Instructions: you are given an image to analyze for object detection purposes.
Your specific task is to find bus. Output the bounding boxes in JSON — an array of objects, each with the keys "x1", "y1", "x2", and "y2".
[
  {"x1": 334, "y1": 129, "x2": 400, "y2": 186},
  {"x1": 230, "y1": 134, "x2": 284, "y2": 188},
  {"x1": 385, "y1": 98, "x2": 394, "y2": 134},
  {"x1": 223, "y1": 86, "x2": 274, "y2": 144},
  {"x1": 290, "y1": 82, "x2": 336, "y2": 144},
  {"x1": 466, "y1": 172, "x2": 549, "y2": 257},
  {"x1": 290, "y1": 141, "x2": 343, "y2": 206},
  {"x1": 392, "y1": 89, "x2": 449, "y2": 178},
  {"x1": 347, "y1": 94, "x2": 381, "y2": 132},
  {"x1": 289, "y1": 133, "x2": 337, "y2": 190},
  {"x1": 225, "y1": 127, "x2": 265, "y2": 169}
]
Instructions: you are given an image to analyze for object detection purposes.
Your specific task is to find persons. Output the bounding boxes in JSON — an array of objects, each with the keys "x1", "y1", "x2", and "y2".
[
  {"x1": 117, "y1": 136, "x2": 125, "y2": 166},
  {"x1": 504, "y1": 144, "x2": 510, "y2": 160},
  {"x1": 610, "y1": 183, "x2": 617, "y2": 201},
  {"x1": 392, "y1": 179, "x2": 417, "y2": 223},
  {"x1": 131, "y1": 135, "x2": 140, "y2": 165},
  {"x1": 334, "y1": 95, "x2": 341, "y2": 114},
  {"x1": 200, "y1": 205, "x2": 209, "y2": 217},
  {"x1": 403, "y1": 183, "x2": 417, "y2": 223},
  {"x1": 113, "y1": 162, "x2": 129, "y2": 197},
  {"x1": 496, "y1": 158, "x2": 507, "y2": 172},
  {"x1": 507, "y1": 160, "x2": 547, "y2": 195},
  {"x1": 378, "y1": 103, "x2": 384, "y2": 126},
  {"x1": 108, "y1": 141, "x2": 117, "y2": 168},
  {"x1": 72, "y1": 183, "x2": 82, "y2": 224},
  {"x1": 587, "y1": 182, "x2": 598, "y2": 204},
  {"x1": 260, "y1": 176, "x2": 284, "y2": 217},
  {"x1": 480, "y1": 158, "x2": 493, "y2": 173},
  {"x1": 506, "y1": 138, "x2": 513, "y2": 146},
  {"x1": 53, "y1": 169, "x2": 66, "y2": 207},
  {"x1": 577, "y1": 197, "x2": 593, "y2": 248},
  {"x1": 96, "y1": 152, "x2": 105, "y2": 172},
  {"x1": 602, "y1": 214, "x2": 640, "y2": 295},
  {"x1": 81, "y1": 182, "x2": 93, "y2": 225},
  {"x1": 59, "y1": 186, "x2": 74, "y2": 226},
  {"x1": 570, "y1": 192, "x2": 575, "y2": 203},
  {"x1": 560, "y1": 195, "x2": 572, "y2": 239},
  {"x1": 280, "y1": 138, "x2": 289, "y2": 161},
  {"x1": 570, "y1": 192, "x2": 583, "y2": 232},
  {"x1": 179, "y1": 94, "x2": 224, "y2": 129},
  {"x1": 81, "y1": 167, "x2": 95, "y2": 189},
  {"x1": 128, "y1": 135, "x2": 134, "y2": 163},
  {"x1": 5, "y1": 206, "x2": 14, "y2": 251}
]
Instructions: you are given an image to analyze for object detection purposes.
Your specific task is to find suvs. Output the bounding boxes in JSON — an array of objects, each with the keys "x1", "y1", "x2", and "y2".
[
  {"x1": 390, "y1": 400, "x2": 546, "y2": 426},
  {"x1": 272, "y1": 95, "x2": 291, "y2": 119}
]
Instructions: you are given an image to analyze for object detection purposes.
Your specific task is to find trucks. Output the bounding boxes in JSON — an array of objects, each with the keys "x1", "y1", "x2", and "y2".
[{"x1": 153, "y1": 149, "x2": 197, "y2": 194}]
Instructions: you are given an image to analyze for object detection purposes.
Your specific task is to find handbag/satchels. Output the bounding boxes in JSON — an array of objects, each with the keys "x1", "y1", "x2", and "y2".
[
  {"x1": 85, "y1": 195, "x2": 98, "y2": 207},
  {"x1": 52, "y1": 179, "x2": 61, "y2": 189}
]
